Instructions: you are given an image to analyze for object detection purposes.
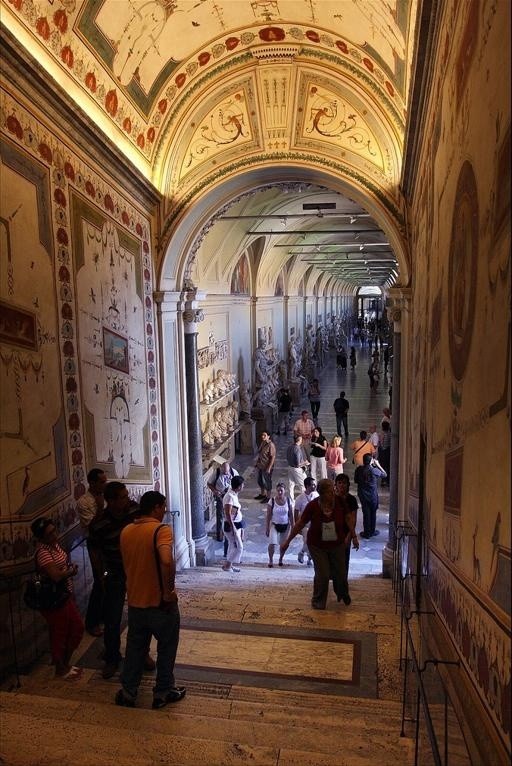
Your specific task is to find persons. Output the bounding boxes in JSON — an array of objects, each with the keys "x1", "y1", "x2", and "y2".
[
  {"x1": 77, "y1": 468, "x2": 107, "y2": 637},
  {"x1": 336, "y1": 317, "x2": 390, "y2": 395},
  {"x1": 198, "y1": 331, "x2": 240, "y2": 445},
  {"x1": 334, "y1": 391, "x2": 349, "y2": 434},
  {"x1": 30, "y1": 516, "x2": 82, "y2": 678},
  {"x1": 241, "y1": 382, "x2": 251, "y2": 421},
  {"x1": 221, "y1": 476, "x2": 244, "y2": 572},
  {"x1": 308, "y1": 379, "x2": 320, "y2": 417},
  {"x1": 254, "y1": 407, "x2": 393, "y2": 610},
  {"x1": 89, "y1": 482, "x2": 155, "y2": 679},
  {"x1": 207, "y1": 463, "x2": 240, "y2": 541},
  {"x1": 277, "y1": 388, "x2": 289, "y2": 435},
  {"x1": 115, "y1": 491, "x2": 186, "y2": 709},
  {"x1": 254, "y1": 327, "x2": 269, "y2": 405}
]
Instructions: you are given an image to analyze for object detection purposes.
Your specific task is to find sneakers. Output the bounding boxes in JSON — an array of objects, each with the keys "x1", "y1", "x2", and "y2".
[
  {"x1": 86, "y1": 623, "x2": 104, "y2": 637},
  {"x1": 115, "y1": 690, "x2": 135, "y2": 708},
  {"x1": 342, "y1": 593, "x2": 351, "y2": 606},
  {"x1": 368, "y1": 530, "x2": 380, "y2": 537},
  {"x1": 152, "y1": 687, "x2": 186, "y2": 707},
  {"x1": 255, "y1": 494, "x2": 266, "y2": 499},
  {"x1": 360, "y1": 532, "x2": 370, "y2": 539},
  {"x1": 102, "y1": 664, "x2": 119, "y2": 679},
  {"x1": 298, "y1": 552, "x2": 312, "y2": 565},
  {"x1": 54, "y1": 665, "x2": 85, "y2": 681},
  {"x1": 221, "y1": 566, "x2": 241, "y2": 573},
  {"x1": 260, "y1": 496, "x2": 270, "y2": 503}
]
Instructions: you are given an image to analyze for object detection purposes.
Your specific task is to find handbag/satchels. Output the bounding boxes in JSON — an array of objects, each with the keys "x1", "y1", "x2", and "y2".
[
  {"x1": 273, "y1": 522, "x2": 289, "y2": 533},
  {"x1": 151, "y1": 601, "x2": 177, "y2": 644},
  {"x1": 23, "y1": 580, "x2": 71, "y2": 612}
]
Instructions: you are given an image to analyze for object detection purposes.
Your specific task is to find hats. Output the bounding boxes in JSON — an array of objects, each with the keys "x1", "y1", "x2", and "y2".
[{"x1": 294, "y1": 433, "x2": 302, "y2": 439}]
